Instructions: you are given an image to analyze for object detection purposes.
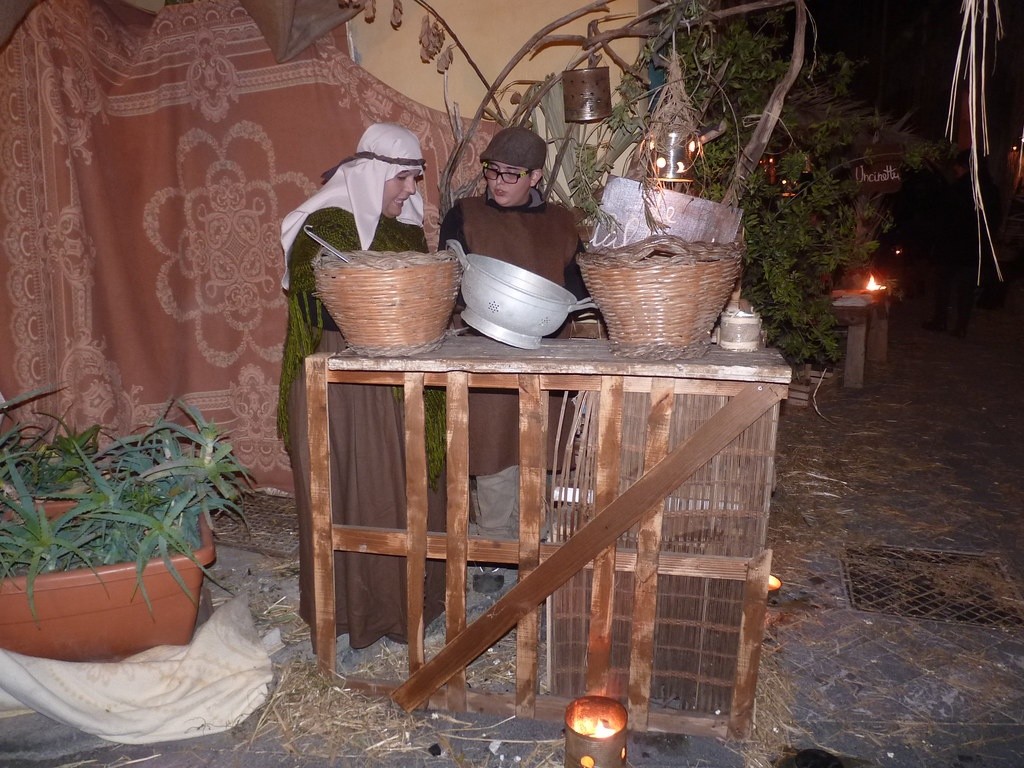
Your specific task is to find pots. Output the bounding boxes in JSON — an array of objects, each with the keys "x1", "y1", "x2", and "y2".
[{"x1": 447, "y1": 238, "x2": 598, "y2": 349}]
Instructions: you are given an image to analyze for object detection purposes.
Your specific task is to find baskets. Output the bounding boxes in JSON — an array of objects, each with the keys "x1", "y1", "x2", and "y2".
[
  {"x1": 310, "y1": 246, "x2": 462, "y2": 357},
  {"x1": 575, "y1": 235, "x2": 741, "y2": 360}
]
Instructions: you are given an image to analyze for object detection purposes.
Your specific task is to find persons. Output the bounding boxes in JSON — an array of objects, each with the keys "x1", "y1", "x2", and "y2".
[
  {"x1": 439, "y1": 126, "x2": 609, "y2": 593},
  {"x1": 276, "y1": 124, "x2": 447, "y2": 647}
]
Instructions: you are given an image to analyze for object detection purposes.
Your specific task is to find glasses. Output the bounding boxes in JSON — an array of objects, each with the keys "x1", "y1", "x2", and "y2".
[{"x1": 482, "y1": 162, "x2": 534, "y2": 184}]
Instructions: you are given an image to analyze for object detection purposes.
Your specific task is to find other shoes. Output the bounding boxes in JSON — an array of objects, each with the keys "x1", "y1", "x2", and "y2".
[{"x1": 472, "y1": 566, "x2": 504, "y2": 592}]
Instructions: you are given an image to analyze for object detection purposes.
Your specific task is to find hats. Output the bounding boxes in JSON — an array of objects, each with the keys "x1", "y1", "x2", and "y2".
[{"x1": 479, "y1": 127, "x2": 547, "y2": 169}]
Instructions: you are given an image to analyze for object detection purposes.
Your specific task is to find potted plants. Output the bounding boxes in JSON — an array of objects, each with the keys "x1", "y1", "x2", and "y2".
[{"x1": 0, "y1": 390, "x2": 257, "y2": 664}]
